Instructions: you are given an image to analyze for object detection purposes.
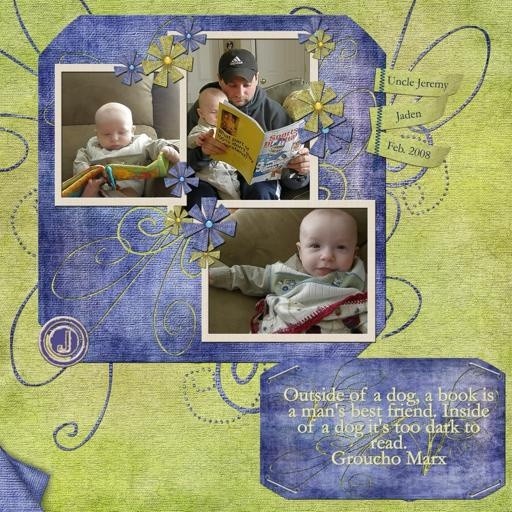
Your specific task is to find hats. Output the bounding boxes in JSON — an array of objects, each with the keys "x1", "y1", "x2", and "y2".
[{"x1": 217, "y1": 48, "x2": 258, "y2": 84}]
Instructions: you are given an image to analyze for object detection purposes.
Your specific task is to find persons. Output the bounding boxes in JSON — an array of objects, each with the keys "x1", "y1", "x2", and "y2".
[
  {"x1": 188, "y1": 86, "x2": 244, "y2": 198},
  {"x1": 70, "y1": 100, "x2": 179, "y2": 198},
  {"x1": 187, "y1": 47, "x2": 310, "y2": 200},
  {"x1": 209, "y1": 207, "x2": 368, "y2": 334}
]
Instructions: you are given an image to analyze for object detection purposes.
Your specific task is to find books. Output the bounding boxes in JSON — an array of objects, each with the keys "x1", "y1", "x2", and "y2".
[
  {"x1": 207, "y1": 99, "x2": 308, "y2": 187},
  {"x1": 61, "y1": 150, "x2": 170, "y2": 197}
]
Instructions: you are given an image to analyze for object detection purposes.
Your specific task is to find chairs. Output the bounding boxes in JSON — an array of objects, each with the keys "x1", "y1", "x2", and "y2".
[{"x1": 187, "y1": 78, "x2": 311, "y2": 200}]
